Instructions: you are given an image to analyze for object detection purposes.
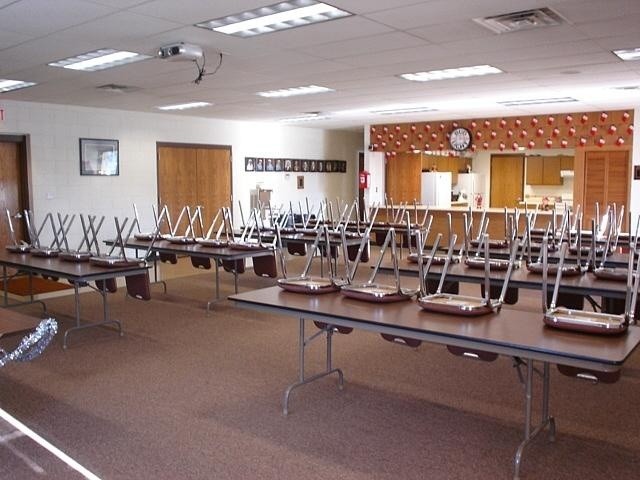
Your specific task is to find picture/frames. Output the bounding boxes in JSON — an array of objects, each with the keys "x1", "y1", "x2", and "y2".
[
  {"x1": 78, "y1": 137, "x2": 121, "y2": 178},
  {"x1": 243, "y1": 156, "x2": 348, "y2": 173}
]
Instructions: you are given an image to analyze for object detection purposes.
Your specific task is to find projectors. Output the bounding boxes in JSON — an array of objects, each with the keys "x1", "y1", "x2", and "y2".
[{"x1": 157, "y1": 42, "x2": 203, "y2": 62}]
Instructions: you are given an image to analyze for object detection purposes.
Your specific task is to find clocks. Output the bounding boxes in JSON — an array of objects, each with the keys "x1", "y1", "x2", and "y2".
[{"x1": 449, "y1": 127, "x2": 472, "y2": 152}]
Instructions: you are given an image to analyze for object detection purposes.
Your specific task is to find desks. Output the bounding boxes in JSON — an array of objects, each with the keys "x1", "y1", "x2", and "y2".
[
  {"x1": 101, "y1": 238, "x2": 276, "y2": 318},
  {"x1": 1, "y1": 250, "x2": 154, "y2": 349},
  {"x1": 225, "y1": 287, "x2": 640, "y2": 479}
]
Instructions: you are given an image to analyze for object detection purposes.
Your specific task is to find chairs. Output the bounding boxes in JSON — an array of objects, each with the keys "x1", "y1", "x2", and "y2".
[
  {"x1": 277, "y1": 194, "x2": 640, "y2": 382},
  {"x1": 2, "y1": 203, "x2": 288, "y2": 301}
]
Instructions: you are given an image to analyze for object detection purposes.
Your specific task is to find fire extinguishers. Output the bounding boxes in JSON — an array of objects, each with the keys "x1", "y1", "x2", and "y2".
[{"x1": 359, "y1": 169, "x2": 370, "y2": 189}]
